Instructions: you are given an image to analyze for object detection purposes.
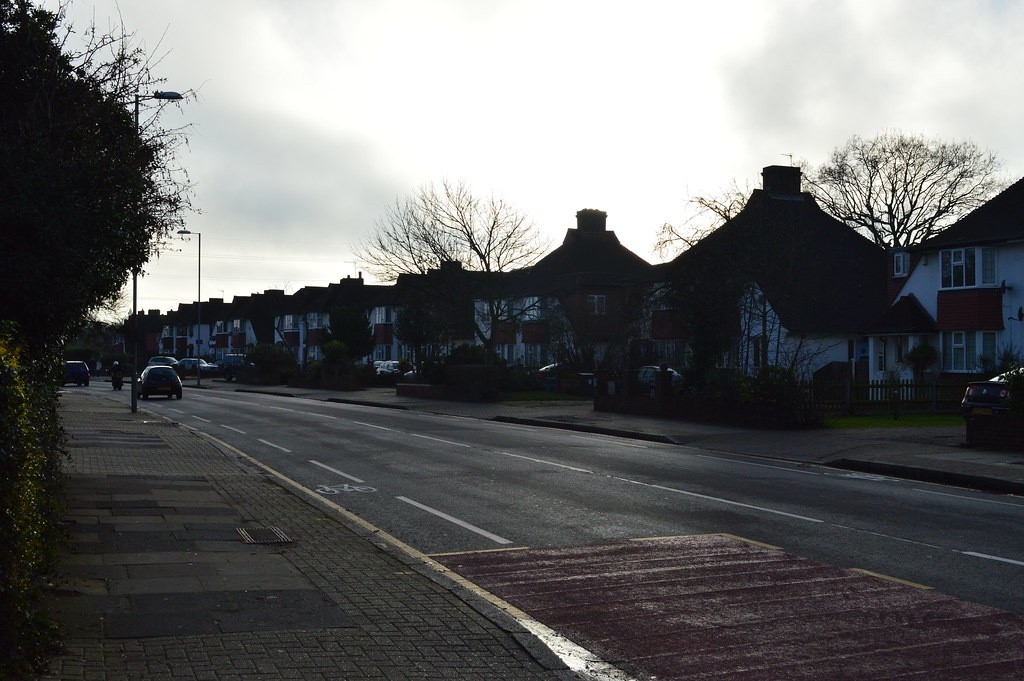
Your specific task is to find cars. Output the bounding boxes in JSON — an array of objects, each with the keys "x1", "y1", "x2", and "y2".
[
  {"x1": 137, "y1": 365, "x2": 183, "y2": 400},
  {"x1": 532, "y1": 362, "x2": 682, "y2": 403},
  {"x1": 961, "y1": 365, "x2": 1024, "y2": 437},
  {"x1": 371, "y1": 358, "x2": 426, "y2": 379}
]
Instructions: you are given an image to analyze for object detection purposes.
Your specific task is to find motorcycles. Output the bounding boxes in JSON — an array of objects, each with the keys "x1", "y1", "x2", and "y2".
[{"x1": 111, "y1": 371, "x2": 124, "y2": 391}]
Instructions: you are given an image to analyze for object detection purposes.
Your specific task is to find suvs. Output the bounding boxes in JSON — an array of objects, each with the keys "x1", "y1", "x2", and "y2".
[
  {"x1": 222, "y1": 353, "x2": 256, "y2": 382},
  {"x1": 148, "y1": 356, "x2": 181, "y2": 376},
  {"x1": 179, "y1": 358, "x2": 219, "y2": 378},
  {"x1": 61, "y1": 360, "x2": 90, "y2": 387}
]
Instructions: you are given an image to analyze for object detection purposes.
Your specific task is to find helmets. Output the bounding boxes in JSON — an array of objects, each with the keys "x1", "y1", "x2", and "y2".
[{"x1": 114, "y1": 361, "x2": 119, "y2": 365}]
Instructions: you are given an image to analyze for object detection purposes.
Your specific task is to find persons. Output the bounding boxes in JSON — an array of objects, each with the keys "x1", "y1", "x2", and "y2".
[{"x1": 107, "y1": 361, "x2": 123, "y2": 386}]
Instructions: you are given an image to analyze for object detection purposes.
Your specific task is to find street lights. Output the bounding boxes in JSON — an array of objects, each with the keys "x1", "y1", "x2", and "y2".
[
  {"x1": 131, "y1": 89, "x2": 184, "y2": 415},
  {"x1": 177, "y1": 230, "x2": 201, "y2": 388}
]
